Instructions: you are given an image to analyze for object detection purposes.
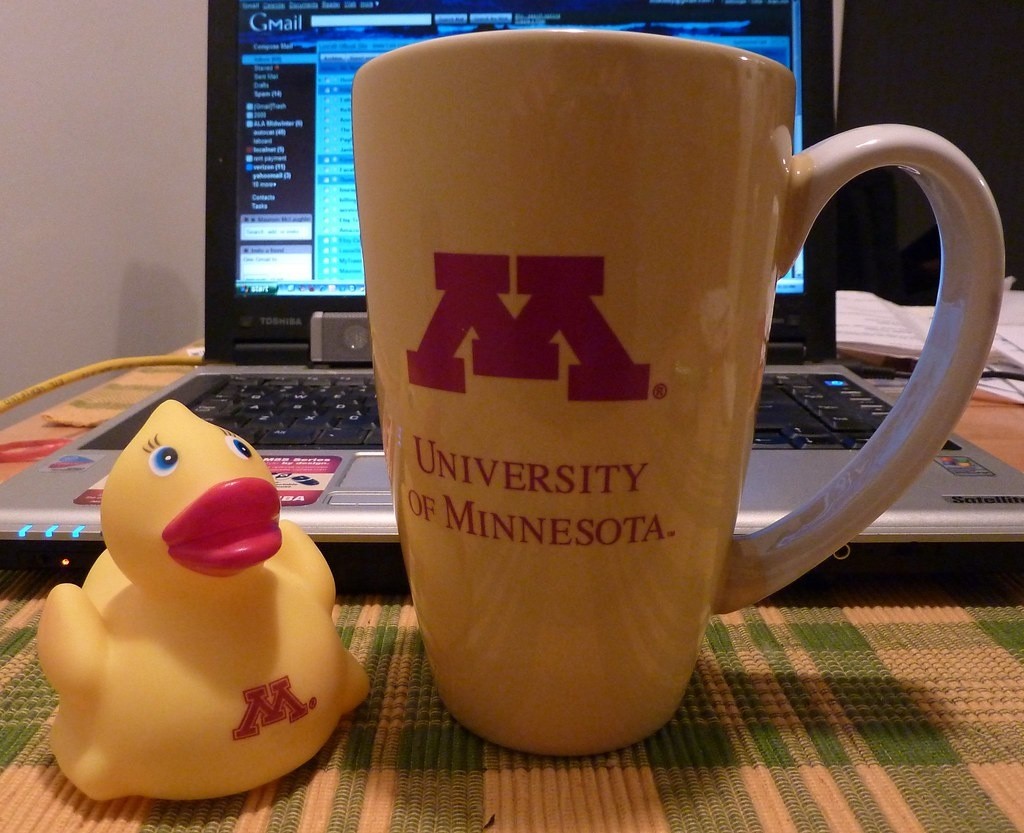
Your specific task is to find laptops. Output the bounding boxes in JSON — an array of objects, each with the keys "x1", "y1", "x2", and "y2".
[{"x1": 0, "y1": 0, "x2": 1024, "y2": 595}]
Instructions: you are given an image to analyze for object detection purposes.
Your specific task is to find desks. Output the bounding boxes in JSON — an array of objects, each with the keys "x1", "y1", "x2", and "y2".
[{"x1": 1, "y1": 337, "x2": 1023, "y2": 832}]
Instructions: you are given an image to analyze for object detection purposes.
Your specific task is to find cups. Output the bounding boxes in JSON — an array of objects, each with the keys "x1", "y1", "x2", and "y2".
[{"x1": 351, "y1": 28, "x2": 1005, "y2": 759}]
